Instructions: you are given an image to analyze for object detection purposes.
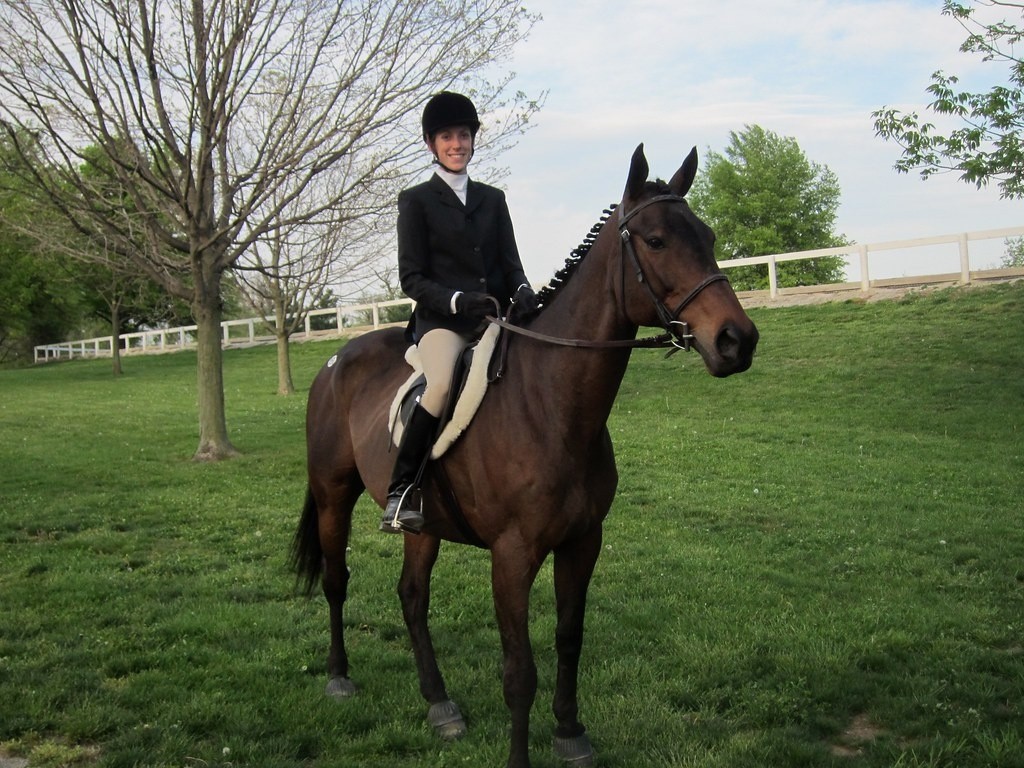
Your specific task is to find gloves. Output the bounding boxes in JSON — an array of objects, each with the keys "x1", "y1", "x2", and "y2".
[
  {"x1": 450, "y1": 290, "x2": 494, "y2": 330},
  {"x1": 509, "y1": 284, "x2": 538, "y2": 311}
]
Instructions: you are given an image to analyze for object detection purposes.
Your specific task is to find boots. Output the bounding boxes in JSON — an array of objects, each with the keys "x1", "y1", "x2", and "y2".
[{"x1": 378, "y1": 399, "x2": 439, "y2": 532}]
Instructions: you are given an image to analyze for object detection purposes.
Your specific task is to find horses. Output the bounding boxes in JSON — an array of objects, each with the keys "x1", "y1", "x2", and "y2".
[{"x1": 283, "y1": 143, "x2": 760, "y2": 768}]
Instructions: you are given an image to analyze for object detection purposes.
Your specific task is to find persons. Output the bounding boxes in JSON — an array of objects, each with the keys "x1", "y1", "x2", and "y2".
[{"x1": 378, "y1": 92, "x2": 536, "y2": 533}]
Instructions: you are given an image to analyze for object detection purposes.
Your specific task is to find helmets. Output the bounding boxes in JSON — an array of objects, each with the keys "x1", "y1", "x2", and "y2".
[{"x1": 423, "y1": 92, "x2": 480, "y2": 142}]
